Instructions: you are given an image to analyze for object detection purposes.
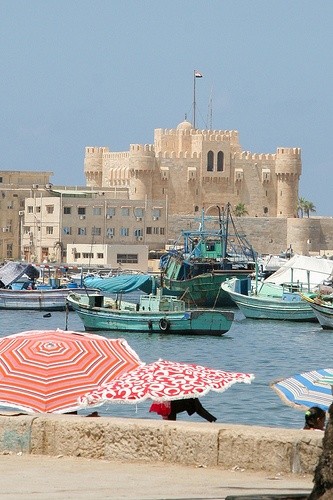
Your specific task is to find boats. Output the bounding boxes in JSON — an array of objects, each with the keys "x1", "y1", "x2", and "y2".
[
  {"x1": 158, "y1": 201, "x2": 261, "y2": 307},
  {"x1": 0, "y1": 260, "x2": 101, "y2": 310},
  {"x1": 221, "y1": 253, "x2": 333, "y2": 321},
  {"x1": 300, "y1": 275, "x2": 333, "y2": 330},
  {"x1": 67, "y1": 285, "x2": 236, "y2": 335}
]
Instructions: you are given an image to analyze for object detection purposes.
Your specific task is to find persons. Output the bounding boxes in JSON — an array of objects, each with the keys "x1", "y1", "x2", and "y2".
[{"x1": 303, "y1": 407, "x2": 327, "y2": 431}]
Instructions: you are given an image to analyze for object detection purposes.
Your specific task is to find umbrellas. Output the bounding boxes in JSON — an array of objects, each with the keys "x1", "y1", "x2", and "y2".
[
  {"x1": 271, "y1": 368, "x2": 333, "y2": 418},
  {"x1": 0, "y1": 328, "x2": 255, "y2": 417}
]
